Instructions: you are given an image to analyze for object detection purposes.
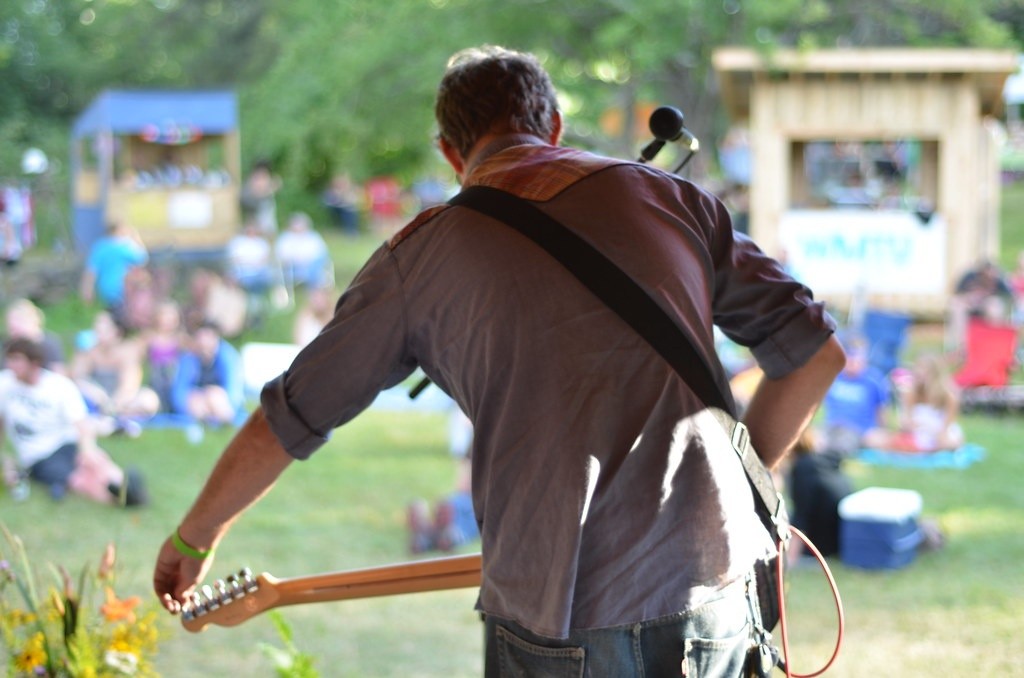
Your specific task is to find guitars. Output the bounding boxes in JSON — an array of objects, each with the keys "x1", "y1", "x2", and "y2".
[{"x1": 181, "y1": 550, "x2": 480, "y2": 633}]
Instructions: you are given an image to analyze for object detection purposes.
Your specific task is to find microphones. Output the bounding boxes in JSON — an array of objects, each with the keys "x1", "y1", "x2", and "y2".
[{"x1": 649, "y1": 104, "x2": 700, "y2": 155}]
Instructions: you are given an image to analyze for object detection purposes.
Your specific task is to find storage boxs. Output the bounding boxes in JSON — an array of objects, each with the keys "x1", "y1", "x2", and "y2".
[{"x1": 838, "y1": 486, "x2": 924, "y2": 570}]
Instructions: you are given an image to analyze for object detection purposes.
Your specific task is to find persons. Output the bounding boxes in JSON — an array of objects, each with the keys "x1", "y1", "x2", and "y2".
[
  {"x1": 152, "y1": 41, "x2": 842, "y2": 676},
  {"x1": 3, "y1": 158, "x2": 445, "y2": 429},
  {"x1": 0, "y1": 340, "x2": 142, "y2": 509},
  {"x1": 827, "y1": 334, "x2": 961, "y2": 453},
  {"x1": 950, "y1": 258, "x2": 1016, "y2": 334}
]
